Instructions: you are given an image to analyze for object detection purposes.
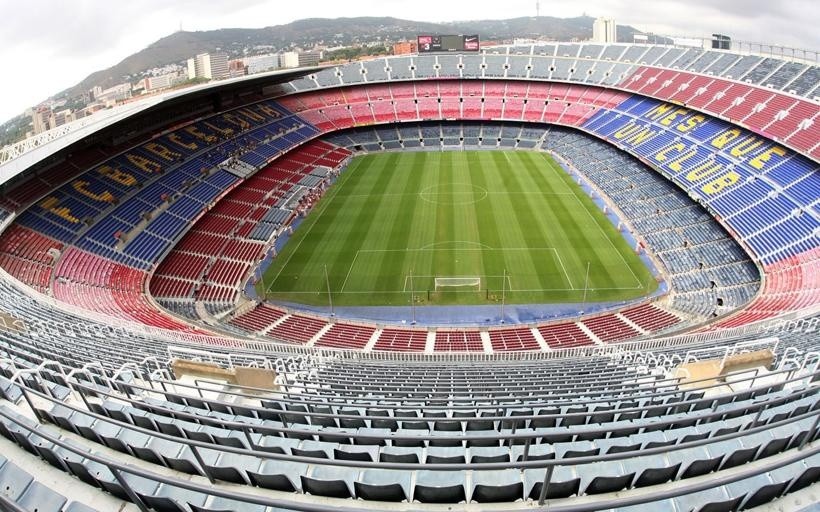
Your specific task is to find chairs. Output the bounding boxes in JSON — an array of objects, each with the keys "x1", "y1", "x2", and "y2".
[{"x1": 1, "y1": 347, "x2": 820, "y2": 512}]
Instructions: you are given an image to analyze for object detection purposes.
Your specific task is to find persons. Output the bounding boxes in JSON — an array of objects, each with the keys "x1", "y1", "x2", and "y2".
[
  {"x1": 206, "y1": 119, "x2": 256, "y2": 170},
  {"x1": 296, "y1": 188, "x2": 324, "y2": 209}
]
